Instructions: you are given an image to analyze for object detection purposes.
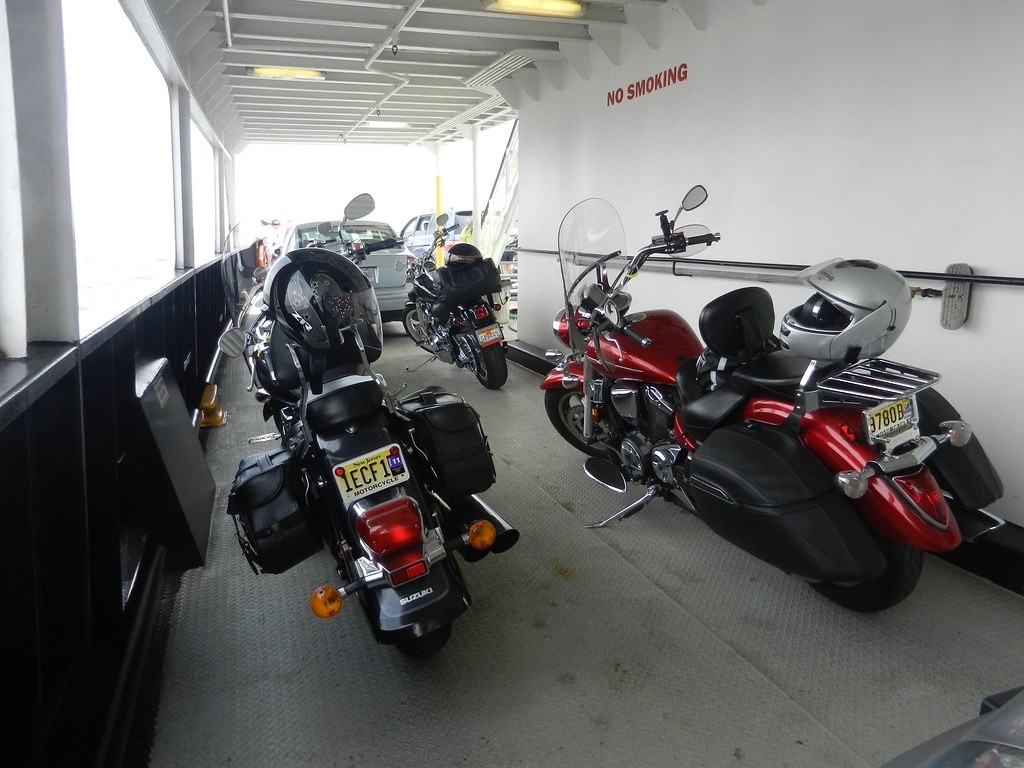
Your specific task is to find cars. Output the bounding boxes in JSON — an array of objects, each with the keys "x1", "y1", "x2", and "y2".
[{"x1": 281, "y1": 220, "x2": 420, "y2": 323}]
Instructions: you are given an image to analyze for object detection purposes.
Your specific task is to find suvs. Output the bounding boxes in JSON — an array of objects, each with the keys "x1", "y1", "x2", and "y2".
[{"x1": 398, "y1": 209, "x2": 518, "y2": 276}]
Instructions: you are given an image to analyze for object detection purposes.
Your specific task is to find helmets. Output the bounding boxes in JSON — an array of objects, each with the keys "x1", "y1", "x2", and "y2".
[
  {"x1": 444, "y1": 243, "x2": 483, "y2": 270},
  {"x1": 262, "y1": 248, "x2": 384, "y2": 363},
  {"x1": 779, "y1": 258, "x2": 913, "y2": 362}
]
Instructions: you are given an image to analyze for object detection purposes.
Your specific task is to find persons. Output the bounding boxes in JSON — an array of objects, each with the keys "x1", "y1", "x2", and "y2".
[
  {"x1": 263, "y1": 218, "x2": 283, "y2": 268},
  {"x1": 458, "y1": 218, "x2": 472, "y2": 243}
]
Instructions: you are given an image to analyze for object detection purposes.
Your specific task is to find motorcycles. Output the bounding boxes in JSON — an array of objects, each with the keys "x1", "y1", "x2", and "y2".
[
  {"x1": 540, "y1": 184, "x2": 1006, "y2": 612},
  {"x1": 393, "y1": 213, "x2": 509, "y2": 390},
  {"x1": 217, "y1": 193, "x2": 522, "y2": 654}
]
